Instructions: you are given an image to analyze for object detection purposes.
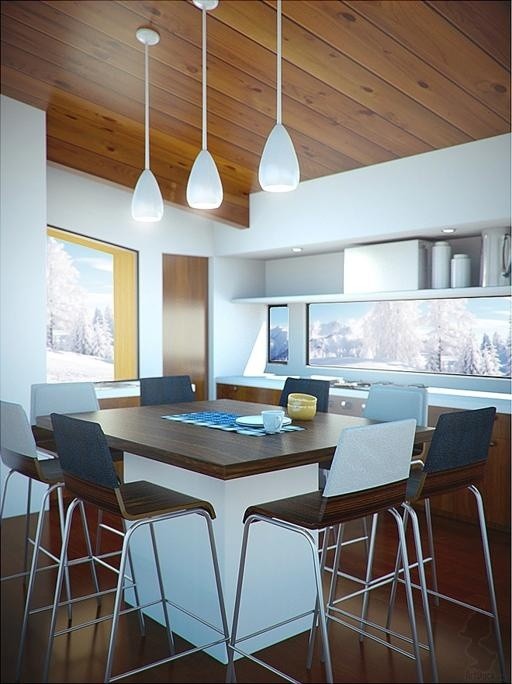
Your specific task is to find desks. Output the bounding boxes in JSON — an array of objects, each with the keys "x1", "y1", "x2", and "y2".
[{"x1": 29, "y1": 395, "x2": 378, "y2": 666}]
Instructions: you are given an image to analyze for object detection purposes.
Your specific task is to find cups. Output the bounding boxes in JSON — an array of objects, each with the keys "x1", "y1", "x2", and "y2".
[
  {"x1": 262, "y1": 410, "x2": 285, "y2": 435},
  {"x1": 479, "y1": 227, "x2": 512, "y2": 286}
]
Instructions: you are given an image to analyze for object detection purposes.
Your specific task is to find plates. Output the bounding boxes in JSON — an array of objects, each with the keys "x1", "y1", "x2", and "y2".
[{"x1": 236, "y1": 415, "x2": 292, "y2": 427}]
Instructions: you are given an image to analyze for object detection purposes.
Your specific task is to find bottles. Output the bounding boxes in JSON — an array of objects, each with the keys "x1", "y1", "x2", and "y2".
[{"x1": 431, "y1": 241, "x2": 471, "y2": 288}]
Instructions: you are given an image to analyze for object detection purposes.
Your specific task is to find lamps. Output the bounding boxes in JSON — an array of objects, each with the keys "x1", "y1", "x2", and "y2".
[
  {"x1": 259, "y1": 1, "x2": 301, "y2": 193},
  {"x1": 186, "y1": 0, "x2": 224, "y2": 209},
  {"x1": 131, "y1": 26, "x2": 164, "y2": 221}
]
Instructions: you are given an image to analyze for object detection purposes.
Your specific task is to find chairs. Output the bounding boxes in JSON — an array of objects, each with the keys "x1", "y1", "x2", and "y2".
[
  {"x1": 138, "y1": 375, "x2": 195, "y2": 406},
  {"x1": 363, "y1": 381, "x2": 429, "y2": 427},
  {"x1": 0, "y1": 400, "x2": 102, "y2": 622},
  {"x1": 277, "y1": 377, "x2": 330, "y2": 415},
  {"x1": 48, "y1": 409, "x2": 229, "y2": 682},
  {"x1": 29, "y1": 380, "x2": 101, "y2": 424},
  {"x1": 383, "y1": 402, "x2": 509, "y2": 680},
  {"x1": 234, "y1": 417, "x2": 426, "y2": 683}
]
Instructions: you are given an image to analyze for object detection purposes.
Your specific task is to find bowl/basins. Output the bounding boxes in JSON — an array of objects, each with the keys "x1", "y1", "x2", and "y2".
[{"x1": 287, "y1": 393, "x2": 318, "y2": 420}]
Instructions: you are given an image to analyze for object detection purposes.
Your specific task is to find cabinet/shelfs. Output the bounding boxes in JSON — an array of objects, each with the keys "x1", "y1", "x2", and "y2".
[
  {"x1": 257, "y1": 299, "x2": 512, "y2": 375},
  {"x1": 207, "y1": 220, "x2": 512, "y2": 306}
]
